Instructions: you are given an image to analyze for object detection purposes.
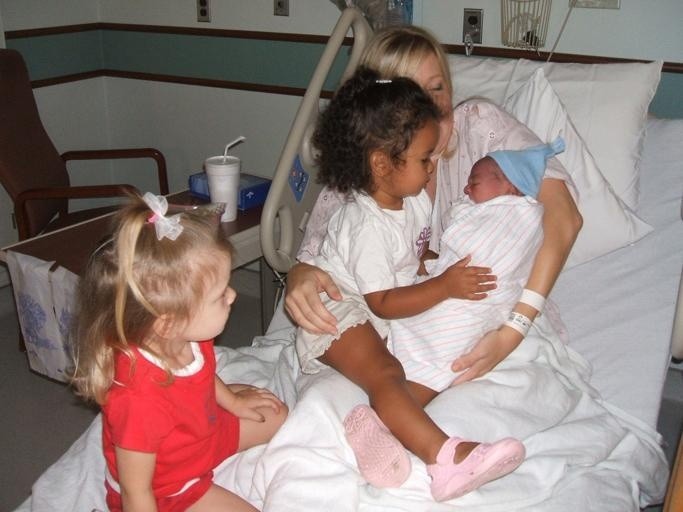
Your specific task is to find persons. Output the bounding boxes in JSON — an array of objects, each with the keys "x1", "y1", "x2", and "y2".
[
  {"x1": 73, "y1": 190, "x2": 288, "y2": 512},
  {"x1": 302, "y1": 70, "x2": 525, "y2": 503},
  {"x1": 277, "y1": 30, "x2": 582, "y2": 409},
  {"x1": 386, "y1": 151, "x2": 539, "y2": 383}
]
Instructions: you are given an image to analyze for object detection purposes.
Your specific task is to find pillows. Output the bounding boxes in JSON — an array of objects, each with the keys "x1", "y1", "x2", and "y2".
[
  {"x1": 498, "y1": 70, "x2": 655, "y2": 271},
  {"x1": 440, "y1": 54, "x2": 665, "y2": 217}
]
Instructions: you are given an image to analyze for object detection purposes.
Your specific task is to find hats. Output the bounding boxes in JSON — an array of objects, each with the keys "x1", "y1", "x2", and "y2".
[{"x1": 485, "y1": 137, "x2": 566, "y2": 199}]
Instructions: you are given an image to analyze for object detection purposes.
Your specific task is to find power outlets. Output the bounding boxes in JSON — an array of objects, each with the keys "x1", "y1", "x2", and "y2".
[{"x1": 461, "y1": 8, "x2": 483, "y2": 44}]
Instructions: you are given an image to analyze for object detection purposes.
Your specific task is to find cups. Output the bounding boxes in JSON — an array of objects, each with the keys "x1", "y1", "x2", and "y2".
[{"x1": 204, "y1": 156, "x2": 240, "y2": 222}]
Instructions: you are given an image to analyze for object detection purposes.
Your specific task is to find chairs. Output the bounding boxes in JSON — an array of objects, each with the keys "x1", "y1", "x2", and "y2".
[{"x1": 1, "y1": 48, "x2": 168, "y2": 354}]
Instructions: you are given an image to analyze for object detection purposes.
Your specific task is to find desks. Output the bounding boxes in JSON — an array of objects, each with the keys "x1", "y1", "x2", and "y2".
[{"x1": 0, "y1": 188, "x2": 266, "y2": 384}]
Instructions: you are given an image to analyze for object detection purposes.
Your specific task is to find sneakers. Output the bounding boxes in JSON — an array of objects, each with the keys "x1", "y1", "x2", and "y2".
[
  {"x1": 426, "y1": 436, "x2": 526, "y2": 502},
  {"x1": 342, "y1": 404, "x2": 411, "y2": 489}
]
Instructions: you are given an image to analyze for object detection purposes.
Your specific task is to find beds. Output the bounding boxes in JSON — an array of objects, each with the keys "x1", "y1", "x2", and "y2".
[{"x1": 15, "y1": 6, "x2": 683, "y2": 511}]
